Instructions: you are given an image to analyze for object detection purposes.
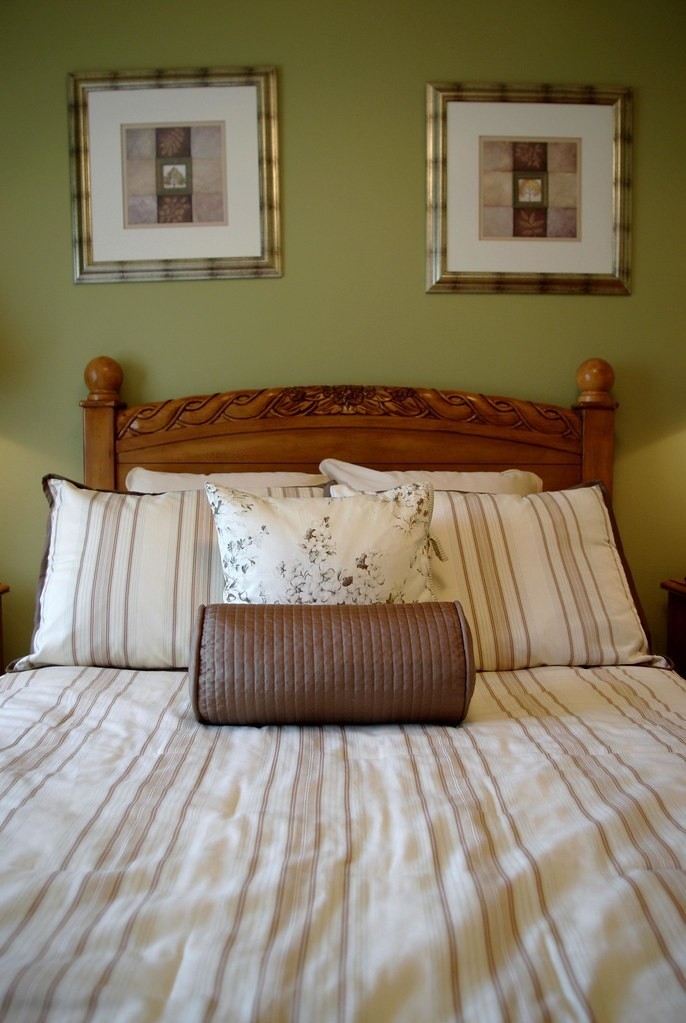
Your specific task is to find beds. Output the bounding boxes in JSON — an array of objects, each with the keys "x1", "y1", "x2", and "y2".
[{"x1": 0, "y1": 353, "x2": 684, "y2": 1023}]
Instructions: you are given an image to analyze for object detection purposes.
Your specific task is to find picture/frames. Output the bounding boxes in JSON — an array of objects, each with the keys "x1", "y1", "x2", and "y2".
[
  {"x1": 62, "y1": 67, "x2": 282, "y2": 283},
  {"x1": 420, "y1": 77, "x2": 635, "y2": 300}
]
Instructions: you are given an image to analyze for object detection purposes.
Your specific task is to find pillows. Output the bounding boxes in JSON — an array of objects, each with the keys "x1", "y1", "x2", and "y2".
[
  {"x1": 206, "y1": 482, "x2": 444, "y2": 609},
  {"x1": 357, "y1": 486, "x2": 674, "y2": 668},
  {"x1": 188, "y1": 597, "x2": 473, "y2": 727},
  {"x1": 315, "y1": 458, "x2": 548, "y2": 496},
  {"x1": 6, "y1": 472, "x2": 337, "y2": 673},
  {"x1": 126, "y1": 467, "x2": 334, "y2": 497}
]
